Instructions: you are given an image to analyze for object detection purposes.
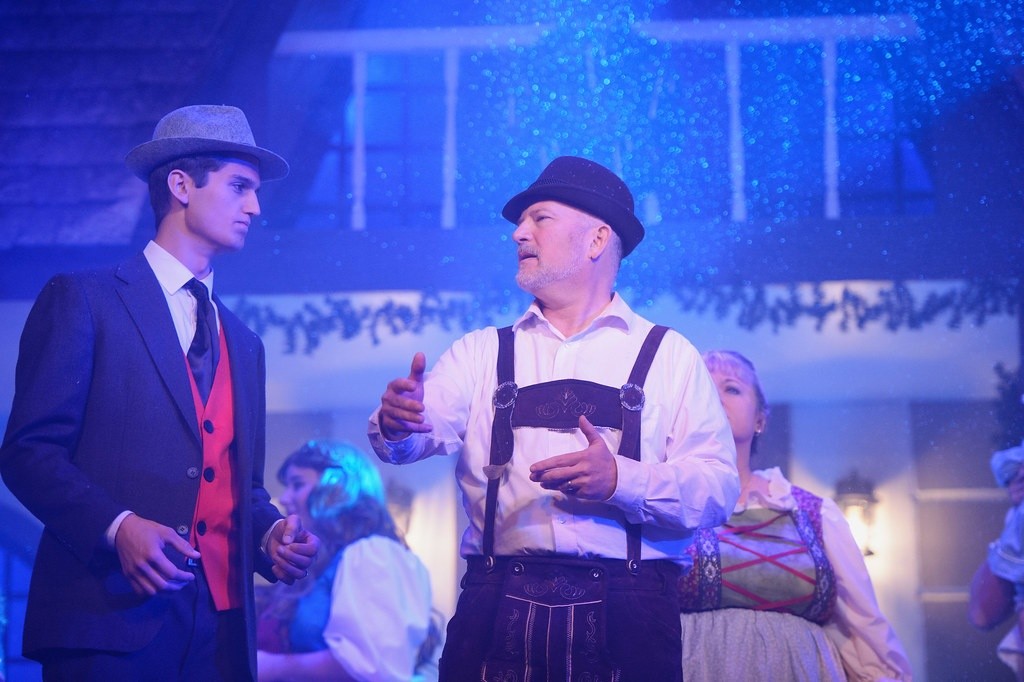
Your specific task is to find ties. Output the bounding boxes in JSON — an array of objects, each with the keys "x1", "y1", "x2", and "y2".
[{"x1": 183, "y1": 277, "x2": 220, "y2": 409}]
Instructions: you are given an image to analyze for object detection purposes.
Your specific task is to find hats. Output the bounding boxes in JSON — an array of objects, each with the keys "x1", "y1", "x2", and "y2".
[
  {"x1": 126, "y1": 104, "x2": 291, "y2": 184},
  {"x1": 502, "y1": 155, "x2": 645, "y2": 258}
]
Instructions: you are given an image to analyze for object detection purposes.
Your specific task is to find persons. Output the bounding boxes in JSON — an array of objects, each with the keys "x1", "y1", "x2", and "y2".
[
  {"x1": 0, "y1": 105, "x2": 291, "y2": 681},
  {"x1": 677, "y1": 349, "x2": 913, "y2": 682},
  {"x1": 257, "y1": 437, "x2": 449, "y2": 682},
  {"x1": 967, "y1": 440, "x2": 1024, "y2": 681},
  {"x1": 365, "y1": 156, "x2": 743, "y2": 682}
]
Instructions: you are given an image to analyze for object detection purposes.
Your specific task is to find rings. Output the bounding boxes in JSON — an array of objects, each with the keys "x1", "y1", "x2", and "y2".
[{"x1": 566, "y1": 480, "x2": 574, "y2": 495}]
